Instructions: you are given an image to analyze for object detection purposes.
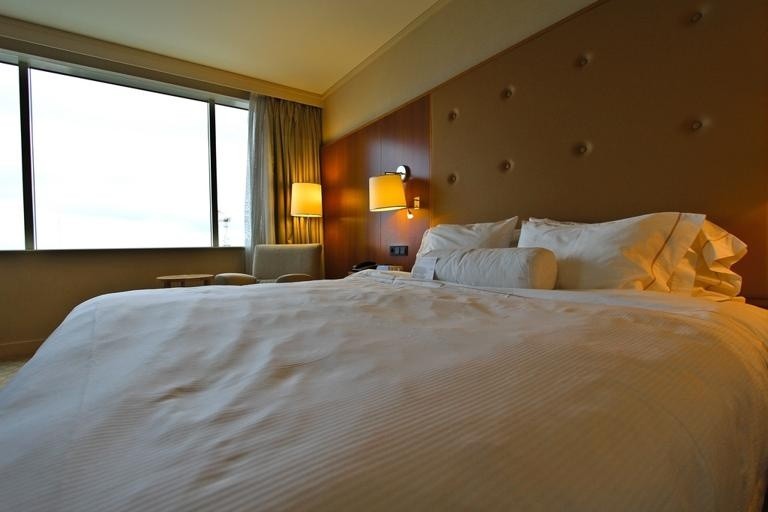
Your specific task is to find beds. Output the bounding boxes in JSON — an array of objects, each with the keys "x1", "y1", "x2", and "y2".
[{"x1": 3, "y1": 270, "x2": 767, "y2": 510}]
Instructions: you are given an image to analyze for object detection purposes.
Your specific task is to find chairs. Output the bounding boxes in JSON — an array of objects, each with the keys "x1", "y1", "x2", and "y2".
[{"x1": 213, "y1": 243, "x2": 321, "y2": 285}]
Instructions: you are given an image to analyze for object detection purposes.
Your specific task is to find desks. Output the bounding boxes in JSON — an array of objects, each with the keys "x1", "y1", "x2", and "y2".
[{"x1": 157, "y1": 273, "x2": 214, "y2": 288}]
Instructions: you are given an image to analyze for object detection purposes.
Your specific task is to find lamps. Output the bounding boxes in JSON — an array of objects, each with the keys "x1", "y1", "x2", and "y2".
[
  {"x1": 368, "y1": 164, "x2": 409, "y2": 212},
  {"x1": 407, "y1": 196, "x2": 420, "y2": 219},
  {"x1": 290, "y1": 182, "x2": 323, "y2": 244}
]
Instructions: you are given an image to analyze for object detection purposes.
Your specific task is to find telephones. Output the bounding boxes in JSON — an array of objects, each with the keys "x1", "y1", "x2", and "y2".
[{"x1": 352, "y1": 260, "x2": 377, "y2": 272}]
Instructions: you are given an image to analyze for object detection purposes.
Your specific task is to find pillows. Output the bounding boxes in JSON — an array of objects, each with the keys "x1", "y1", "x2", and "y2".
[
  {"x1": 529, "y1": 216, "x2": 748, "y2": 302},
  {"x1": 516, "y1": 216, "x2": 709, "y2": 289},
  {"x1": 415, "y1": 215, "x2": 521, "y2": 261},
  {"x1": 419, "y1": 248, "x2": 558, "y2": 290}
]
